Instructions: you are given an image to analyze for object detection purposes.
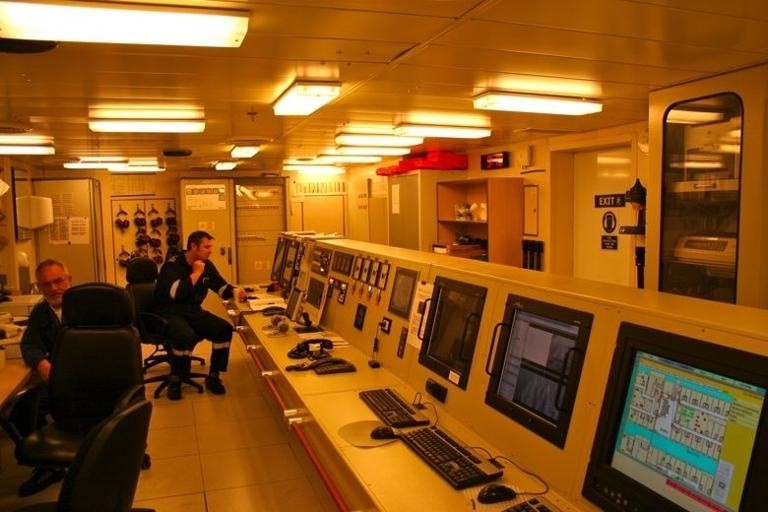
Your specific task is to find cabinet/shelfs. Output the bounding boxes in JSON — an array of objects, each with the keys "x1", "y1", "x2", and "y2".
[{"x1": 437, "y1": 176, "x2": 524, "y2": 269}]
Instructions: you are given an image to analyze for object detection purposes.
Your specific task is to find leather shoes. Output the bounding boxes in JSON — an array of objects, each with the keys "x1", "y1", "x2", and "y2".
[
  {"x1": 206, "y1": 382, "x2": 225, "y2": 395},
  {"x1": 19, "y1": 464, "x2": 63, "y2": 497},
  {"x1": 167, "y1": 381, "x2": 182, "y2": 400}
]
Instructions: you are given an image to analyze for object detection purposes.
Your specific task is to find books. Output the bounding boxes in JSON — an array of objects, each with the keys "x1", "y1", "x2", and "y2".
[{"x1": 245, "y1": 292, "x2": 288, "y2": 312}]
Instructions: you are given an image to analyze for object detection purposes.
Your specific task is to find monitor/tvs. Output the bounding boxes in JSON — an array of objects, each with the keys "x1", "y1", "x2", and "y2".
[
  {"x1": 286, "y1": 287, "x2": 303, "y2": 321},
  {"x1": 270, "y1": 235, "x2": 288, "y2": 277},
  {"x1": 387, "y1": 267, "x2": 418, "y2": 320},
  {"x1": 280, "y1": 239, "x2": 300, "y2": 292},
  {"x1": 580, "y1": 320, "x2": 768, "y2": 512},
  {"x1": 417, "y1": 275, "x2": 488, "y2": 392},
  {"x1": 306, "y1": 277, "x2": 324, "y2": 309},
  {"x1": 484, "y1": 293, "x2": 593, "y2": 450}
]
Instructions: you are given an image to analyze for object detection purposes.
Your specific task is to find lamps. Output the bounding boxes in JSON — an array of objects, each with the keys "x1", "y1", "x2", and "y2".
[{"x1": 1, "y1": 1, "x2": 604, "y2": 174}]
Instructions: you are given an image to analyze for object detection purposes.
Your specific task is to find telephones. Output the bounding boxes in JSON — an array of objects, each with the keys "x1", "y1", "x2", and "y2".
[
  {"x1": 263, "y1": 307, "x2": 285, "y2": 316},
  {"x1": 310, "y1": 356, "x2": 356, "y2": 375}
]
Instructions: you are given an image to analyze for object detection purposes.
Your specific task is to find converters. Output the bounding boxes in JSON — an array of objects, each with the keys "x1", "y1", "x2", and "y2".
[{"x1": 367, "y1": 359, "x2": 380, "y2": 368}]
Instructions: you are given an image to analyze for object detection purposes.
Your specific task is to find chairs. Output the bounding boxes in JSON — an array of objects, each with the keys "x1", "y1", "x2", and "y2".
[
  {"x1": 122, "y1": 259, "x2": 210, "y2": 399},
  {"x1": 29, "y1": 401, "x2": 152, "y2": 510},
  {"x1": 1, "y1": 282, "x2": 151, "y2": 480}
]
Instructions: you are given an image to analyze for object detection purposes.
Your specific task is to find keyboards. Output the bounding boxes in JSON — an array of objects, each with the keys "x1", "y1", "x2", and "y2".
[
  {"x1": 399, "y1": 425, "x2": 503, "y2": 490},
  {"x1": 359, "y1": 388, "x2": 430, "y2": 428},
  {"x1": 500, "y1": 495, "x2": 561, "y2": 512}
]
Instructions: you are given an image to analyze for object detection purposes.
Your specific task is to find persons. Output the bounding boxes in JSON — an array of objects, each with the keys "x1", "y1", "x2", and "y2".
[
  {"x1": 154, "y1": 230, "x2": 247, "y2": 399},
  {"x1": 7, "y1": 260, "x2": 151, "y2": 497}
]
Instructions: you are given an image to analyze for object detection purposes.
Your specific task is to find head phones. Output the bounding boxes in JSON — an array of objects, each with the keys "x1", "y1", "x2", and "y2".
[{"x1": 262, "y1": 314, "x2": 288, "y2": 336}]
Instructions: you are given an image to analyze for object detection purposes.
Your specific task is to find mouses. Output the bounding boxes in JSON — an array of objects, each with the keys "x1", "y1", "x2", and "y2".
[
  {"x1": 477, "y1": 483, "x2": 516, "y2": 505},
  {"x1": 371, "y1": 426, "x2": 403, "y2": 440}
]
